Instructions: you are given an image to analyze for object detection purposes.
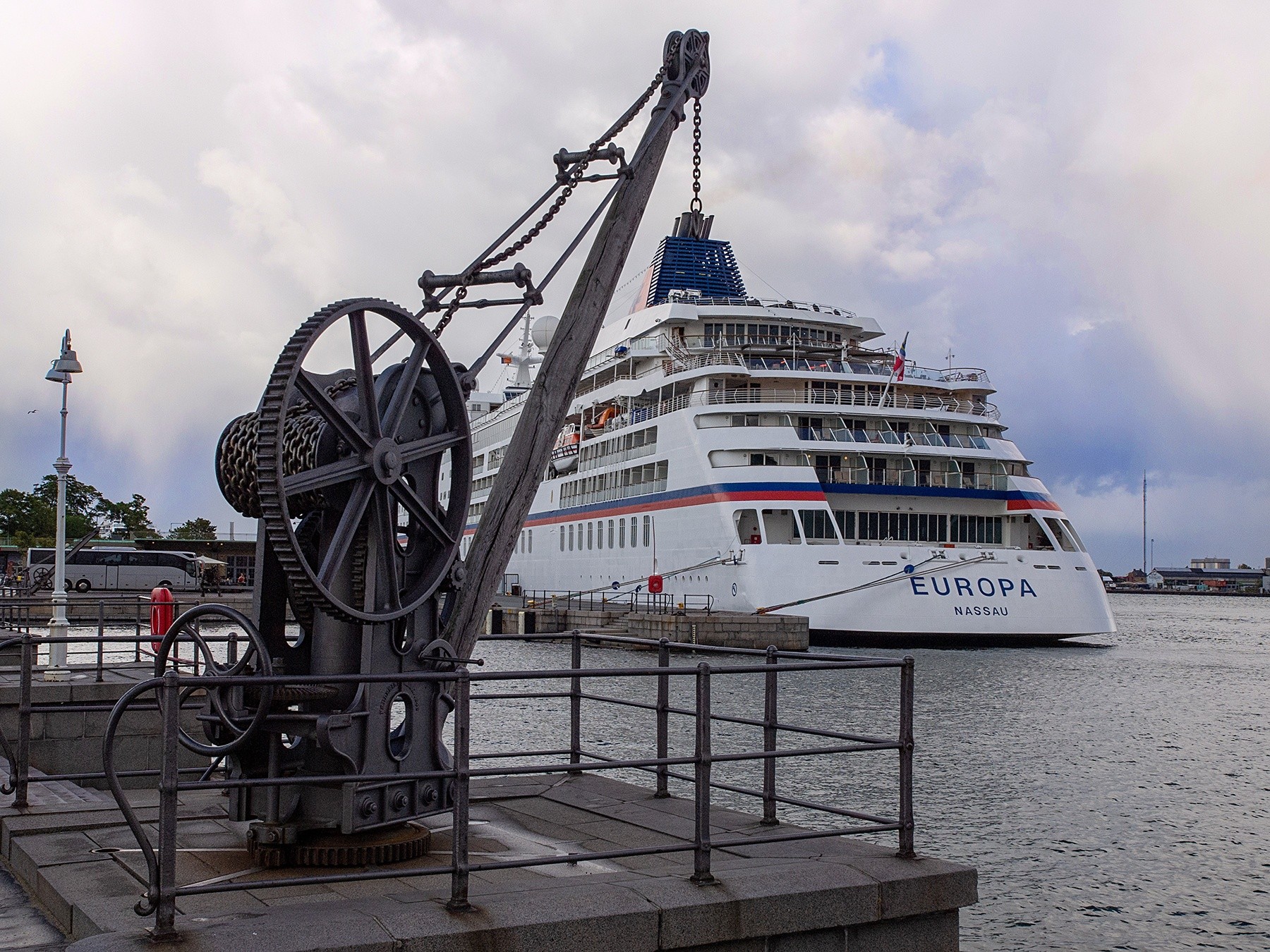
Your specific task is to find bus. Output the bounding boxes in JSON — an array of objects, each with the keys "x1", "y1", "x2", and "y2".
[{"x1": 27, "y1": 547, "x2": 205, "y2": 594}]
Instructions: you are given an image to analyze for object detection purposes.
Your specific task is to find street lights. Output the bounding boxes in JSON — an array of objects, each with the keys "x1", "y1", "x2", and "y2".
[
  {"x1": 96, "y1": 513, "x2": 107, "y2": 528},
  {"x1": 44, "y1": 329, "x2": 82, "y2": 682},
  {"x1": 1151, "y1": 539, "x2": 1154, "y2": 570}
]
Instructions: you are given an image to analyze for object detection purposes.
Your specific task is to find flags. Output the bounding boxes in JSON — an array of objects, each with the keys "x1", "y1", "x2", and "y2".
[{"x1": 893, "y1": 337, "x2": 908, "y2": 382}]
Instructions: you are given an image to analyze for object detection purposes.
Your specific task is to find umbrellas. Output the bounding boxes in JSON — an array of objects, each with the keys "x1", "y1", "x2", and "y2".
[{"x1": 196, "y1": 556, "x2": 228, "y2": 568}]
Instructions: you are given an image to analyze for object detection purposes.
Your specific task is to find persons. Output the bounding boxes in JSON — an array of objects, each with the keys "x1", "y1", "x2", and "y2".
[
  {"x1": 226, "y1": 570, "x2": 231, "y2": 585},
  {"x1": 7, "y1": 561, "x2": 15, "y2": 585},
  {"x1": 238, "y1": 573, "x2": 246, "y2": 591},
  {"x1": 101, "y1": 558, "x2": 152, "y2": 565}
]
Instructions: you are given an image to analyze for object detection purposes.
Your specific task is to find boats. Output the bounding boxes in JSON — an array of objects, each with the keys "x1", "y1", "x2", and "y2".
[
  {"x1": 587, "y1": 408, "x2": 630, "y2": 437},
  {"x1": 549, "y1": 426, "x2": 581, "y2": 473}
]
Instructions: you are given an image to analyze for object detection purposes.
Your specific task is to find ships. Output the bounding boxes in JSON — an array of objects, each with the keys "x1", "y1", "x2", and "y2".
[{"x1": 399, "y1": 212, "x2": 1117, "y2": 644}]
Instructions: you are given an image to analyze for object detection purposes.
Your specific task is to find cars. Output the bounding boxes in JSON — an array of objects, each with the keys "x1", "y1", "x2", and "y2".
[
  {"x1": 1132, "y1": 582, "x2": 1149, "y2": 589},
  {"x1": 1101, "y1": 576, "x2": 1113, "y2": 584}
]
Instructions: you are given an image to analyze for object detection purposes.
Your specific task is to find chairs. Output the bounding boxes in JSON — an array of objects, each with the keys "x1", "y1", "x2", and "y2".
[
  {"x1": 926, "y1": 475, "x2": 945, "y2": 488},
  {"x1": 771, "y1": 364, "x2": 780, "y2": 370},
  {"x1": 941, "y1": 405, "x2": 949, "y2": 411},
  {"x1": 834, "y1": 471, "x2": 856, "y2": 484},
  {"x1": 887, "y1": 472, "x2": 899, "y2": 485},
  {"x1": 813, "y1": 431, "x2": 830, "y2": 440},
  {"x1": 963, "y1": 477, "x2": 977, "y2": 489}
]
